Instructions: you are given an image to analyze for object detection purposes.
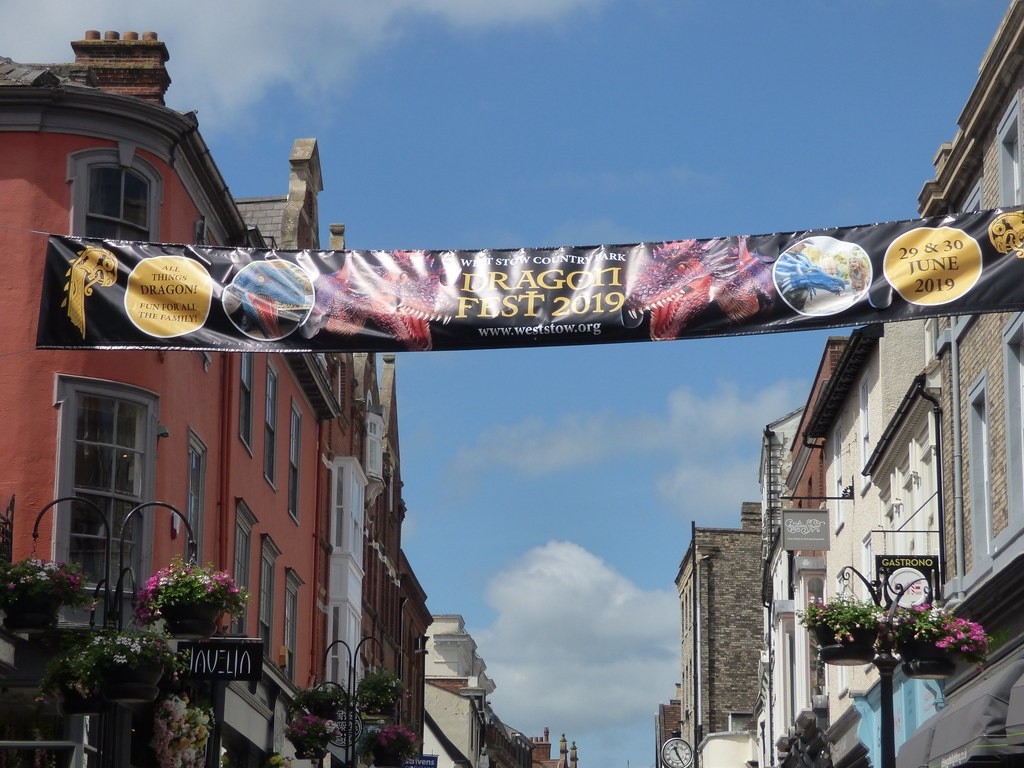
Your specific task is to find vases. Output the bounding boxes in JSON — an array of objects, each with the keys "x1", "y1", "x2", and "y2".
[
  {"x1": 899, "y1": 647, "x2": 955, "y2": 679},
  {"x1": 57, "y1": 689, "x2": 112, "y2": 716},
  {"x1": 287, "y1": 733, "x2": 331, "y2": 759},
  {"x1": 371, "y1": 748, "x2": 405, "y2": 768},
  {"x1": 97, "y1": 660, "x2": 165, "y2": 704},
  {"x1": 814, "y1": 625, "x2": 879, "y2": 666},
  {"x1": 311, "y1": 702, "x2": 338, "y2": 722},
  {"x1": 159, "y1": 601, "x2": 225, "y2": 641},
  {"x1": 363, "y1": 696, "x2": 400, "y2": 715},
  {"x1": 3, "y1": 593, "x2": 63, "y2": 633}
]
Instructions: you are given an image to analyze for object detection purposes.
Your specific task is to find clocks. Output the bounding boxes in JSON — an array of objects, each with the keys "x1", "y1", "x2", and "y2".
[{"x1": 660, "y1": 737, "x2": 693, "y2": 768}]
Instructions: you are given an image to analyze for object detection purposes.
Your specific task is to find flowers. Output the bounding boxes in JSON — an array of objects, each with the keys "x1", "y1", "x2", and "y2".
[
  {"x1": 0, "y1": 553, "x2": 100, "y2": 614},
  {"x1": 790, "y1": 593, "x2": 899, "y2": 655},
  {"x1": 356, "y1": 718, "x2": 422, "y2": 766},
  {"x1": 287, "y1": 683, "x2": 356, "y2": 721},
  {"x1": 131, "y1": 554, "x2": 253, "y2": 630},
  {"x1": 219, "y1": 751, "x2": 237, "y2": 768},
  {"x1": 33, "y1": 626, "x2": 191, "y2": 705},
  {"x1": 266, "y1": 749, "x2": 294, "y2": 768},
  {"x1": 148, "y1": 689, "x2": 216, "y2": 768},
  {"x1": 356, "y1": 669, "x2": 412, "y2": 715},
  {"x1": 890, "y1": 601, "x2": 1006, "y2": 672},
  {"x1": 282, "y1": 713, "x2": 342, "y2": 765}
]
